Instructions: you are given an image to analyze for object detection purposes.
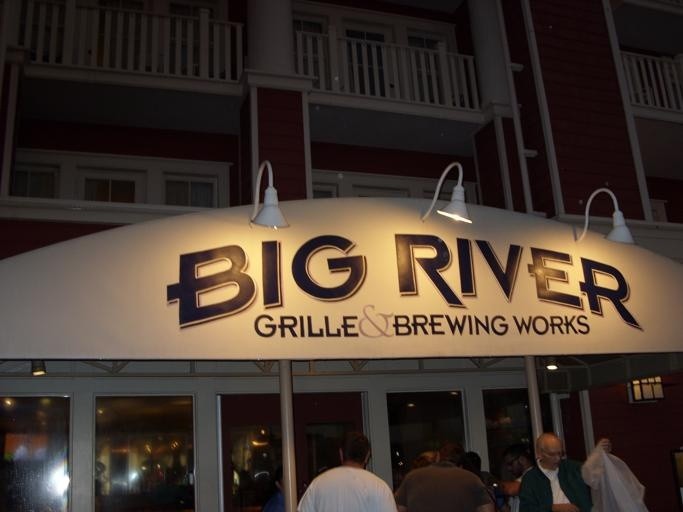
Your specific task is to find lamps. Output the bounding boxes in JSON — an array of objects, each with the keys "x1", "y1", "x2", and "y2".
[
  {"x1": 575, "y1": 187, "x2": 636, "y2": 245},
  {"x1": 250, "y1": 157, "x2": 290, "y2": 230}
]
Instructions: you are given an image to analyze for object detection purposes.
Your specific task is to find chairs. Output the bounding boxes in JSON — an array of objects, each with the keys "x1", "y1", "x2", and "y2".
[{"x1": 421, "y1": 161, "x2": 474, "y2": 227}]
